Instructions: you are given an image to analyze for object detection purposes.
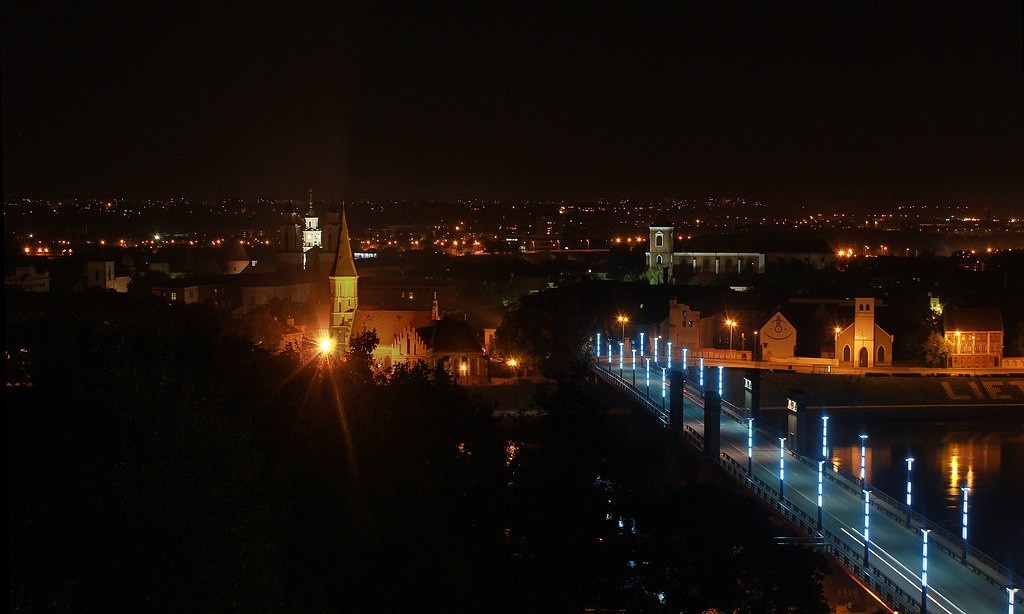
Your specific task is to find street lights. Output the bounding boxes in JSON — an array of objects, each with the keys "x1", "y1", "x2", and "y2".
[
  {"x1": 618, "y1": 316, "x2": 628, "y2": 342},
  {"x1": 726, "y1": 319, "x2": 737, "y2": 350},
  {"x1": 835, "y1": 327, "x2": 841, "y2": 358}
]
